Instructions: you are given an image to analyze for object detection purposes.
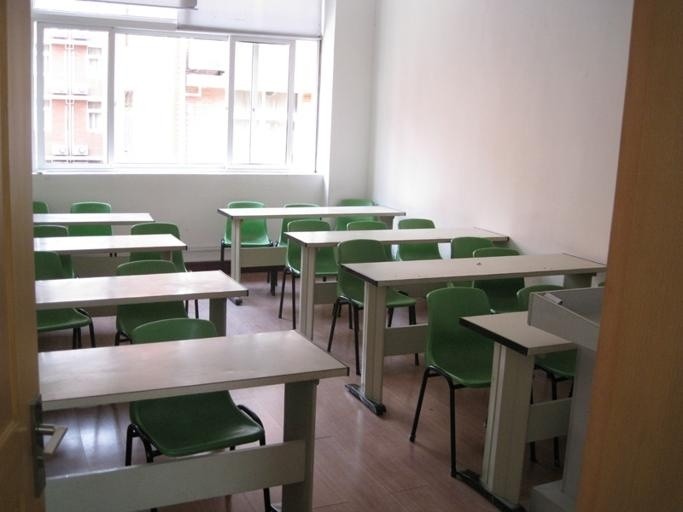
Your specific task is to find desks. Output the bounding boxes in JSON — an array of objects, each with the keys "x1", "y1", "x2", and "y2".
[
  {"x1": 454, "y1": 309, "x2": 576, "y2": 512},
  {"x1": 38, "y1": 329, "x2": 349, "y2": 512}
]
